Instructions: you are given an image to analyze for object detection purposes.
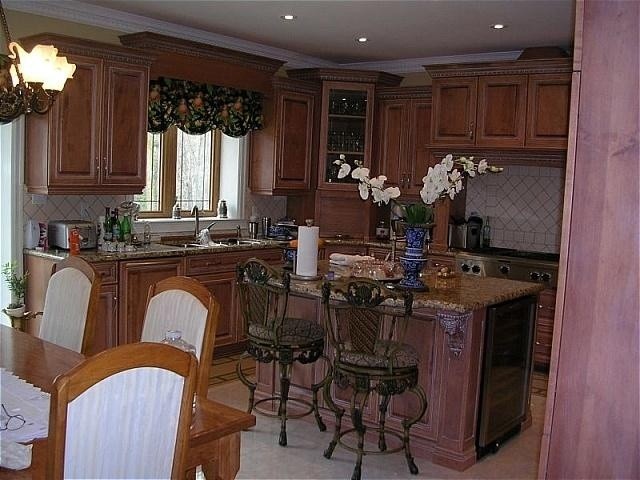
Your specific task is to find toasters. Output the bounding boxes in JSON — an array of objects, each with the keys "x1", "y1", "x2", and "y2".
[{"x1": 48, "y1": 218, "x2": 97, "y2": 251}]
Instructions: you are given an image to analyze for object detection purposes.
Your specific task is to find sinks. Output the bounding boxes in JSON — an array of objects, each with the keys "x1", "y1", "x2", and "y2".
[{"x1": 215, "y1": 240, "x2": 264, "y2": 246}]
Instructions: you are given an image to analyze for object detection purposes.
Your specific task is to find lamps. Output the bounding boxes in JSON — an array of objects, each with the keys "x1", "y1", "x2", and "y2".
[{"x1": 1, "y1": 7, "x2": 82, "y2": 130}]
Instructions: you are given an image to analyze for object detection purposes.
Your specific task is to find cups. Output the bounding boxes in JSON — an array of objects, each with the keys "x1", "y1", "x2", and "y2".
[
  {"x1": 262, "y1": 217, "x2": 272, "y2": 239},
  {"x1": 325, "y1": 92, "x2": 367, "y2": 182},
  {"x1": 355, "y1": 257, "x2": 403, "y2": 280},
  {"x1": 248, "y1": 221, "x2": 258, "y2": 240}
]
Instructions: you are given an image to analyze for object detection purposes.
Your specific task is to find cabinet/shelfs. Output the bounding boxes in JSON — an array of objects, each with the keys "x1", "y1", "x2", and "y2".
[
  {"x1": 385, "y1": 193, "x2": 467, "y2": 252},
  {"x1": 523, "y1": 58, "x2": 578, "y2": 154},
  {"x1": 286, "y1": 188, "x2": 391, "y2": 241},
  {"x1": 371, "y1": 244, "x2": 403, "y2": 263},
  {"x1": 422, "y1": 58, "x2": 529, "y2": 156},
  {"x1": 381, "y1": 83, "x2": 467, "y2": 198},
  {"x1": 533, "y1": 0, "x2": 640, "y2": 479},
  {"x1": 423, "y1": 249, "x2": 455, "y2": 272},
  {"x1": 311, "y1": 65, "x2": 380, "y2": 189},
  {"x1": 534, "y1": 288, "x2": 556, "y2": 371}
]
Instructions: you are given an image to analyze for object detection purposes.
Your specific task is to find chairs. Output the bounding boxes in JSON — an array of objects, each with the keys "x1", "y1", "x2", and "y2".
[
  {"x1": 312, "y1": 272, "x2": 432, "y2": 480},
  {"x1": 45, "y1": 337, "x2": 205, "y2": 478},
  {"x1": 228, "y1": 250, "x2": 328, "y2": 447},
  {"x1": 135, "y1": 273, "x2": 221, "y2": 398}
]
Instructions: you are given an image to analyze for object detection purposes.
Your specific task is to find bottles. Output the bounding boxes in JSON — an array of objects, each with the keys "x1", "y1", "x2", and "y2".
[
  {"x1": 144, "y1": 221, "x2": 150, "y2": 245},
  {"x1": 69, "y1": 227, "x2": 81, "y2": 254},
  {"x1": 100, "y1": 204, "x2": 134, "y2": 253},
  {"x1": 481, "y1": 216, "x2": 491, "y2": 250},
  {"x1": 217, "y1": 199, "x2": 227, "y2": 218},
  {"x1": 154, "y1": 327, "x2": 198, "y2": 428},
  {"x1": 37, "y1": 221, "x2": 48, "y2": 250},
  {"x1": 376, "y1": 220, "x2": 389, "y2": 243},
  {"x1": 172, "y1": 203, "x2": 181, "y2": 219}
]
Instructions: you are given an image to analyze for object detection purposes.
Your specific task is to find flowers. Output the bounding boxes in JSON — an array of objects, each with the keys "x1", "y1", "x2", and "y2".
[{"x1": 326, "y1": 146, "x2": 506, "y2": 225}]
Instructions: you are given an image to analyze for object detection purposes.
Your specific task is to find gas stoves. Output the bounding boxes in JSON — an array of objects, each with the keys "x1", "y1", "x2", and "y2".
[{"x1": 460, "y1": 247, "x2": 559, "y2": 291}]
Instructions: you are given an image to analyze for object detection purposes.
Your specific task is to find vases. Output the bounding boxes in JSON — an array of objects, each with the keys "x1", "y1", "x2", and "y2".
[{"x1": 395, "y1": 219, "x2": 434, "y2": 292}]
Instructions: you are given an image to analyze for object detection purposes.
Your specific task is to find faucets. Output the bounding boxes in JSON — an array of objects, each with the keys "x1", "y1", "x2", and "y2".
[
  {"x1": 236, "y1": 225, "x2": 240, "y2": 238},
  {"x1": 191, "y1": 206, "x2": 216, "y2": 241}
]
